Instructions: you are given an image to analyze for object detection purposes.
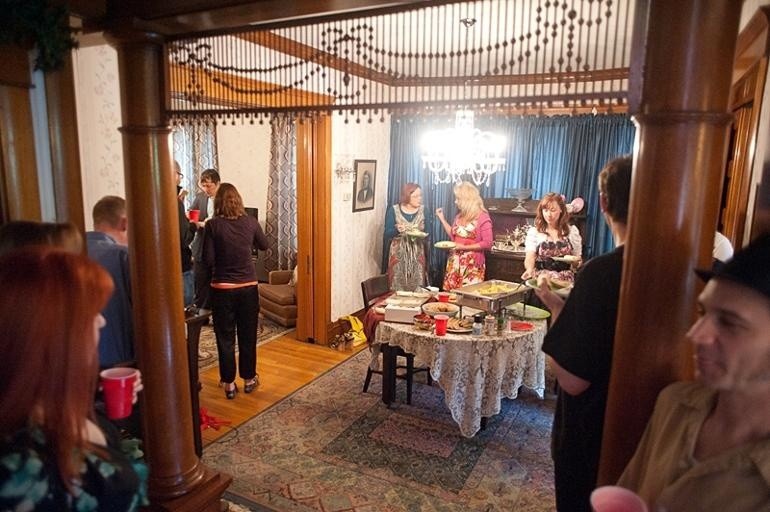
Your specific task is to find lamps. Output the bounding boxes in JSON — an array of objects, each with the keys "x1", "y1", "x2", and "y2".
[
  {"x1": 334, "y1": 162, "x2": 357, "y2": 202},
  {"x1": 415, "y1": 17, "x2": 509, "y2": 189}
]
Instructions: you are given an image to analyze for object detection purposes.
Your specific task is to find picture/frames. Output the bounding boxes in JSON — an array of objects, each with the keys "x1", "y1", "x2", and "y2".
[{"x1": 351, "y1": 158, "x2": 378, "y2": 215}]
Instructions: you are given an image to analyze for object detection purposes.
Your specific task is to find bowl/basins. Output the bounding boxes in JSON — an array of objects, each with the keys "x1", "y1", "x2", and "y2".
[
  {"x1": 422, "y1": 286, "x2": 439, "y2": 297},
  {"x1": 422, "y1": 302, "x2": 460, "y2": 318},
  {"x1": 413, "y1": 315, "x2": 432, "y2": 329}
]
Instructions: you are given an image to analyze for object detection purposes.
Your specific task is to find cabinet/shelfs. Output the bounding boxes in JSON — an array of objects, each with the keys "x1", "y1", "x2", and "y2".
[{"x1": 481, "y1": 196, "x2": 592, "y2": 288}]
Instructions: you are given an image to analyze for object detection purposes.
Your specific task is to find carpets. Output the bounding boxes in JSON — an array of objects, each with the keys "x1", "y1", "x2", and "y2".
[
  {"x1": 183, "y1": 312, "x2": 295, "y2": 375},
  {"x1": 199, "y1": 346, "x2": 556, "y2": 512}
]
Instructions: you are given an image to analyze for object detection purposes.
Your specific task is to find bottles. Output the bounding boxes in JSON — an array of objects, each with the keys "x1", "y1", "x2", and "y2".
[
  {"x1": 497, "y1": 309, "x2": 506, "y2": 329},
  {"x1": 485, "y1": 316, "x2": 495, "y2": 336},
  {"x1": 472, "y1": 315, "x2": 483, "y2": 337}
]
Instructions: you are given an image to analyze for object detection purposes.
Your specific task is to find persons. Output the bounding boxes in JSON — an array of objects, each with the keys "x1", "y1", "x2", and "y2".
[
  {"x1": 382, "y1": 181, "x2": 431, "y2": 292},
  {"x1": 199, "y1": 182, "x2": 269, "y2": 399},
  {"x1": 616, "y1": 225, "x2": 770, "y2": 512},
  {"x1": 357, "y1": 170, "x2": 373, "y2": 203},
  {"x1": 175, "y1": 160, "x2": 202, "y2": 305},
  {"x1": 533, "y1": 152, "x2": 631, "y2": 511},
  {"x1": 434, "y1": 181, "x2": 494, "y2": 292},
  {"x1": 0, "y1": 195, "x2": 153, "y2": 512},
  {"x1": 188, "y1": 168, "x2": 222, "y2": 326},
  {"x1": 520, "y1": 193, "x2": 584, "y2": 311}
]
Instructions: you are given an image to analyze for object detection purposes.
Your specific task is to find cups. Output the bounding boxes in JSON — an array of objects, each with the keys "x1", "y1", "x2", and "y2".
[
  {"x1": 99, "y1": 367, "x2": 138, "y2": 420},
  {"x1": 434, "y1": 314, "x2": 449, "y2": 336},
  {"x1": 438, "y1": 293, "x2": 450, "y2": 301},
  {"x1": 190, "y1": 209, "x2": 200, "y2": 222},
  {"x1": 495, "y1": 235, "x2": 508, "y2": 249},
  {"x1": 510, "y1": 232, "x2": 522, "y2": 252}
]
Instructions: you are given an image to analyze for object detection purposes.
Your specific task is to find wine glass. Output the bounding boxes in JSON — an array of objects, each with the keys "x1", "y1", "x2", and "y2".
[{"x1": 566, "y1": 204, "x2": 576, "y2": 214}]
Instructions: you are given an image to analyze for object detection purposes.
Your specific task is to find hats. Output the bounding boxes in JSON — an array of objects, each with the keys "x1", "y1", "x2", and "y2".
[{"x1": 694, "y1": 230, "x2": 770, "y2": 297}]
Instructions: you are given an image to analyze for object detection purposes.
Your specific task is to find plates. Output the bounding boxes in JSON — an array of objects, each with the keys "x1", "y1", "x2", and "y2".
[
  {"x1": 551, "y1": 256, "x2": 582, "y2": 262},
  {"x1": 511, "y1": 305, "x2": 551, "y2": 319},
  {"x1": 571, "y1": 197, "x2": 585, "y2": 212},
  {"x1": 511, "y1": 321, "x2": 534, "y2": 331},
  {"x1": 433, "y1": 240, "x2": 456, "y2": 249},
  {"x1": 446, "y1": 327, "x2": 473, "y2": 333}
]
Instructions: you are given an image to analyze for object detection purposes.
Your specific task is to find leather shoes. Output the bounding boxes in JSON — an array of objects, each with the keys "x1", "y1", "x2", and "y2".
[
  {"x1": 226, "y1": 382, "x2": 237, "y2": 399},
  {"x1": 245, "y1": 378, "x2": 257, "y2": 393}
]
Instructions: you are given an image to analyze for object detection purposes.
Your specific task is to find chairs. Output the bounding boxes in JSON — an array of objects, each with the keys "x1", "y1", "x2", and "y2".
[
  {"x1": 257, "y1": 269, "x2": 298, "y2": 329},
  {"x1": 359, "y1": 273, "x2": 433, "y2": 406}
]
